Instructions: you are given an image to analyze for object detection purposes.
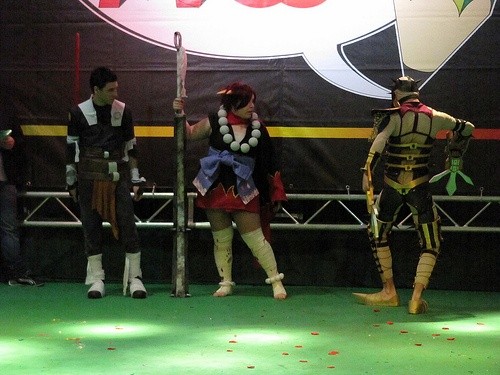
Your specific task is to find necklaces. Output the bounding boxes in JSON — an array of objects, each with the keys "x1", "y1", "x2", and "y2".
[{"x1": 218, "y1": 105, "x2": 261, "y2": 153}]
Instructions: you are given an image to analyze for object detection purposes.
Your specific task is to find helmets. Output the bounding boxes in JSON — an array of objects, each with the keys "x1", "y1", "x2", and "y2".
[{"x1": 387, "y1": 75, "x2": 421, "y2": 108}]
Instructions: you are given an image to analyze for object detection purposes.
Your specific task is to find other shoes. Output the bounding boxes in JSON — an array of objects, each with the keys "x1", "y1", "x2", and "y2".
[
  {"x1": 408, "y1": 297, "x2": 428, "y2": 314},
  {"x1": 353, "y1": 289, "x2": 399, "y2": 307},
  {"x1": 87, "y1": 279, "x2": 105, "y2": 297},
  {"x1": 129, "y1": 278, "x2": 147, "y2": 298}
]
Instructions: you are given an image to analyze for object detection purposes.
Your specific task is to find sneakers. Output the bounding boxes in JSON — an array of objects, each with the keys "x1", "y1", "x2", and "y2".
[{"x1": 8, "y1": 270, "x2": 46, "y2": 287}]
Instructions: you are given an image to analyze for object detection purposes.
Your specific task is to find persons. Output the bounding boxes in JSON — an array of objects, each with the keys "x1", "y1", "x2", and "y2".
[
  {"x1": 65, "y1": 67, "x2": 148, "y2": 298},
  {"x1": 173, "y1": 83, "x2": 288, "y2": 298},
  {"x1": 351, "y1": 75, "x2": 475, "y2": 314},
  {"x1": 0, "y1": 99, "x2": 45, "y2": 288}
]
